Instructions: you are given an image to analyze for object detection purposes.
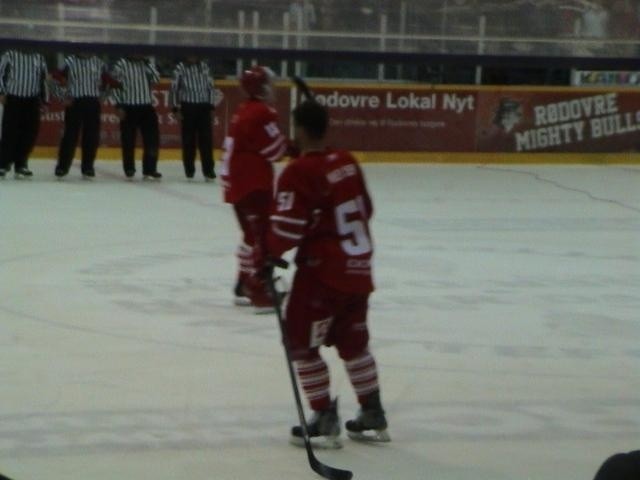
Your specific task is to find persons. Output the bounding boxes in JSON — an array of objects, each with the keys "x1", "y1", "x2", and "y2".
[
  {"x1": 54, "y1": 45, "x2": 113, "y2": 178},
  {"x1": 1, "y1": 44, "x2": 58, "y2": 178},
  {"x1": 170, "y1": 50, "x2": 217, "y2": 181},
  {"x1": 220, "y1": 64, "x2": 294, "y2": 315},
  {"x1": 110, "y1": 51, "x2": 164, "y2": 180},
  {"x1": 248, "y1": 97, "x2": 393, "y2": 450}
]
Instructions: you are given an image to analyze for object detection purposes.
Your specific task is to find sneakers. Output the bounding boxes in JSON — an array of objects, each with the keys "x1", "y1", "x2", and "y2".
[
  {"x1": 82, "y1": 167, "x2": 95, "y2": 177},
  {"x1": 55, "y1": 164, "x2": 67, "y2": 176},
  {"x1": 255, "y1": 289, "x2": 285, "y2": 305},
  {"x1": 344, "y1": 410, "x2": 388, "y2": 431},
  {"x1": 142, "y1": 168, "x2": 161, "y2": 177},
  {"x1": 204, "y1": 169, "x2": 216, "y2": 178},
  {"x1": 186, "y1": 168, "x2": 195, "y2": 177},
  {"x1": 0, "y1": 165, "x2": 8, "y2": 175},
  {"x1": 291, "y1": 400, "x2": 340, "y2": 438},
  {"x1": 125, "y1": 166, "x2": 135, "y2": 177},
  {"x1": 14, "y1": 166, "x2": 32, "y2": 175},
  {"x1": 235, "y1": 279, "x2": 251, "y2": 298}
]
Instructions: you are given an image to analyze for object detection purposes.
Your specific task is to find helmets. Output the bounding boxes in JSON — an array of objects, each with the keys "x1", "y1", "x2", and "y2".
[{"x1": 240, "y1": 67, "x2": 274, "y2": 97}]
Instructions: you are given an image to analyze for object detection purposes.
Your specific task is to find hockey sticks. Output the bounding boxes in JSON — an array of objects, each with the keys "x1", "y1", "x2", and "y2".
[
  {"x1": 268, "y1": 270, "x2": 353, "y2": 480},
  {"x1": 276, "y1": 77, "x2": 313, "y2": 161}
]
